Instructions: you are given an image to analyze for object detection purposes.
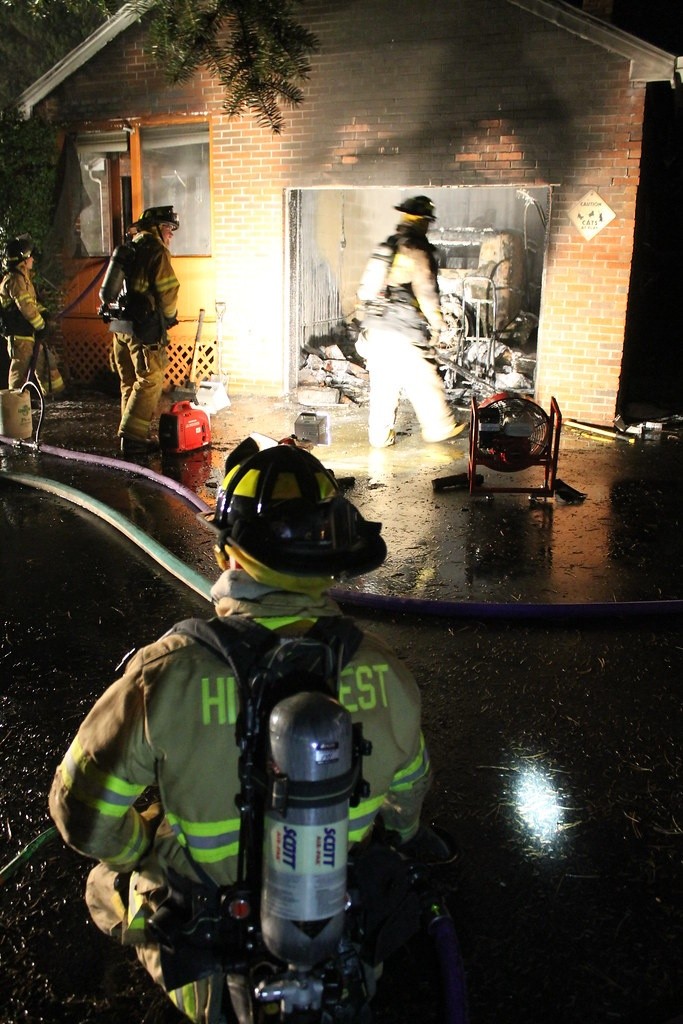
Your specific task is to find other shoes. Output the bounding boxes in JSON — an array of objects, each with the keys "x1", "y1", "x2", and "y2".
[
  {"x1": 116, "y1": 429, "x2": 160, "y2": 446},
  {"x1": 120, "y1": 440, "x2": 156, "y2": 452},
  {"x1": 424, "y1": 422, "x2": 468, "y2": 443},
  {"x1": 375, "y1": 429, "x2": 395, "y2": 446},
  {"x1": 46, "y1": 389, "x2": 77, "y2": 401}
]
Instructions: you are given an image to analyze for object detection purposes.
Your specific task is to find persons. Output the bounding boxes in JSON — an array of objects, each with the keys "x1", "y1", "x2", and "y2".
[
  {"x1": 109, "y1": 204, "x2": 184, "y2": 454},
  {"x1": 0, "y1": 232, "x2": 68, "y2": 398},
  {"x1": 353, "y1": 195, "x2": 468, "y2": 448},
  {"x1": 47, "y1": 433, "x2": 429, "y2": 1023}
]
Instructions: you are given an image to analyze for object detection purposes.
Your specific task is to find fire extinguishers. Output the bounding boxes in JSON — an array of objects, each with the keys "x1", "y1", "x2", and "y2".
[
  {"x1": 357, "y1": 234, "x2": 396, "y2": 299},
  {"x1": 257, "y1": 693, "x2": 353, "y2": 973},
  {"x1": 96, "y1": 240, "x2": 133, "y2": 315}
]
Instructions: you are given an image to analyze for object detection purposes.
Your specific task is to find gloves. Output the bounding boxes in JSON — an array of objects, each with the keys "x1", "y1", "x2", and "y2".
[
  {"x1": 40, "y1": 309, "x2": 49, "y2": 321},
  {"x1": 35, "y1": 325, "x2": 49, "y2": 342},
  {"x1": 140, "y1": 801, "x2": 163, "y2": 834}
]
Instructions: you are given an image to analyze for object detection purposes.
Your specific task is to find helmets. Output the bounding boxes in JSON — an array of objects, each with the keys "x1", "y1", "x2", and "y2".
[
  {"x1": 2, "y1": 239, "x2": 33, "y2": 268},
  {"x1": 213, "y1": 445, "x2": 387, "y2": 578},
  {"x1": 137, "y1": 206, "x2": 179, "y2": 231},
  {"x1": 394, "y1": 196, "x2": 437, "y2": 219}
]
